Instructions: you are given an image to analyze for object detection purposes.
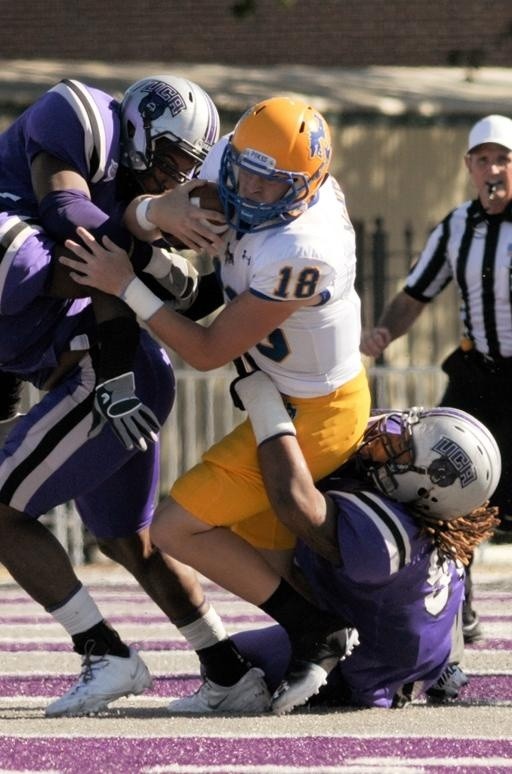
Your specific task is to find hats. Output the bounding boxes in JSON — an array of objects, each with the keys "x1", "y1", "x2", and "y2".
[{"x1": 464, "y1": 112, "x2": 511, "y2": 157}]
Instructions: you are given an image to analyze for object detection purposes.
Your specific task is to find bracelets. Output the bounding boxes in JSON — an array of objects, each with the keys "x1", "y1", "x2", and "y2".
[
  {"x1": 130, "y1": 194, "x2": 160, "y2": 234},
  {"x1": 117, "y1": 277, "x2": 167, "y2": 322}
]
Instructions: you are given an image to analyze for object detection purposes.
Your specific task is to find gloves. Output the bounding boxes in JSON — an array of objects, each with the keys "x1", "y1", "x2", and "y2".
[
  {"x1": 228, "y1": 368, "x2": 298, "y2": 446},
  {"x1": 86, "y1": 370, "x2": 163, "y2": 454},
  {"x1": 141, "y1": 242, "x2": 202, "y2": 312}
]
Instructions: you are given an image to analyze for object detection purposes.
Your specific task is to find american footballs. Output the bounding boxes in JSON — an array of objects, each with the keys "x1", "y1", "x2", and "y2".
[{"x1": 154, "y1": 181, "x2": 236, "y2": 250}]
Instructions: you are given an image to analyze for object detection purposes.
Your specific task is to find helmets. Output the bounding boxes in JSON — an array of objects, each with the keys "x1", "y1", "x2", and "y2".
[
  {"x1": 217, "y1": 95, "x2": 334, "y2": 233},
  {"x1": 122, "y1": 74, "x2": 224, "y2": 169},
  {"x1": 351, "y1": 405, "x2": 502, "y2": 522}
]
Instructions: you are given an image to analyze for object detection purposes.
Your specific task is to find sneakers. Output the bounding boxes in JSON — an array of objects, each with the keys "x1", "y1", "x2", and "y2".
[
  {"x1": 427, "y1": 612, "x2": 483, "y2": 701},
  {"x1": 41, "y1": 636, "x2": 153, "y2": 717},
  {"x1": 271, "y1": 606, "x2": 362, "y2": 715},
  {"x1": 163, "y1": 660, "x2": 271, "y2": 714}
]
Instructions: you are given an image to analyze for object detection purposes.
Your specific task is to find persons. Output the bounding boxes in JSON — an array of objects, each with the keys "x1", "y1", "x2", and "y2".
[
  {"x1": 2, "y1": 75, "x2": 220, "y2": 421},
  {"x1": 359, "y1": 112, "x2": 512, "y2": 643},
  {"x1": 3, "y1": 189, "x2": 276, "y2": 717},
  {"x1": 58, "y1": 92, "x2": 371, "y2": 712},
  {"x1": 198, "y1": 366, "x2": 503, "y2": 715}
]
